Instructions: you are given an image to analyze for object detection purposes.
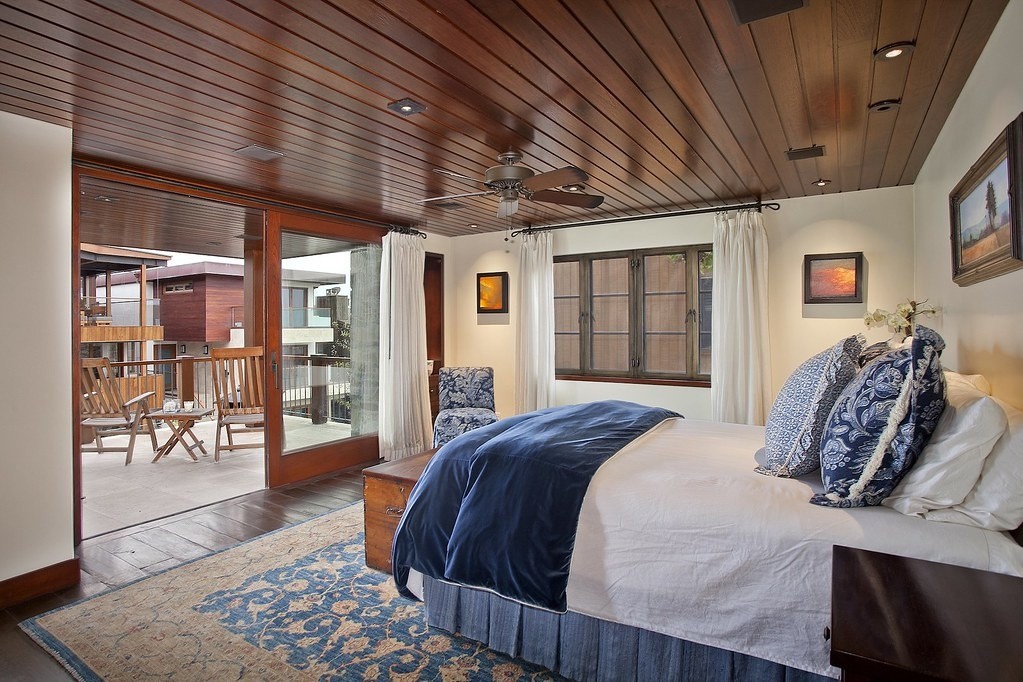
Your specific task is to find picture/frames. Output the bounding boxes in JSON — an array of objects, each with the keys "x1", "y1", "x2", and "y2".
[
  {"x1": 804, "y1": 252, "x2": 863, "y2": 305},
  {"x1": 949, "y1": 112, "x2": 1023, "y2": 288},
  {"x1": 477, "y1": 272, "x2": 508, "y2": 314}
]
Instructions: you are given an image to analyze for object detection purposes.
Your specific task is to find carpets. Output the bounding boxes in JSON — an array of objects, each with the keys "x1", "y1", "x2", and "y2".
[{"x1": 17, "y1": 498, "x2": 581, "y2": 682}]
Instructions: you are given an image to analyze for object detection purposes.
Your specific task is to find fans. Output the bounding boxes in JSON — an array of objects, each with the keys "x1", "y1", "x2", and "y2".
[{"x1": 413, "y1": 151, "x2": 604, "y2": 242}]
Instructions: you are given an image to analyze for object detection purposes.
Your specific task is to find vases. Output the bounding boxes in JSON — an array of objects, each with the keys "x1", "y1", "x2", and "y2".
[{"x1": 890, "y1": 332, "x2": 907, "y2": 343}]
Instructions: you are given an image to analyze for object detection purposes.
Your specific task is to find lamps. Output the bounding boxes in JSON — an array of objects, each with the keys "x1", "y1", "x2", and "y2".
[
  {"x1": 203, "y1": 345, "x2": 208, "y2": 355},
  {"x1": 181, "y1": 345, "x2": 186, "y2": 353}
]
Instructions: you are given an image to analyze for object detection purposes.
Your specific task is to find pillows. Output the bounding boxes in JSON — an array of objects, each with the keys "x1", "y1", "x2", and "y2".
[
  {"x1": 753, "y1": 333, "x2": 866, "y2": 478},
  {"x1": 879, "y1": 371, "x2": 1008, "y2": 521},
  {"x1": 809, "y1": 323, "x2": 948, "y2": 517},
  {"x1": 922, "y1": 395, "x2": 1023, "y2": 531},
  {"x1": 943, "y1": 366, "x2": 991, "y2": 396}
]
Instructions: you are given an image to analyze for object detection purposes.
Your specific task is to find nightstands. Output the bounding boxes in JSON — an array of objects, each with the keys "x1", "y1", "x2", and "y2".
[{"x1": 825, "y1": 545, "x2": 1023, "y2": 682}]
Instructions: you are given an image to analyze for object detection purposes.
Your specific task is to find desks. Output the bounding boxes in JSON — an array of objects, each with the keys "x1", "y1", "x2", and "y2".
[
  {"x1": 88, "y1": 316, "x2": 114, "y2": 326},
  {"x1": 146, "y1": 408, "x2": 216, "y2": 463}
]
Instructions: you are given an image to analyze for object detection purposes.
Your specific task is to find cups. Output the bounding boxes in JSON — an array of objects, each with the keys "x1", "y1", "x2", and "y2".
[
  {"x1": 163, "y1": 398, "x2": 194, "y2": 413},
  {"x1": 427, "y1": 360, "x2": 434, "y2": 376}
]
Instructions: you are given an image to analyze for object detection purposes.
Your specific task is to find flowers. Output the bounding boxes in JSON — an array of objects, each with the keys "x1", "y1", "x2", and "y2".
[{"x1": 863, "y1": 298, "x2": 937, "y2": 333}]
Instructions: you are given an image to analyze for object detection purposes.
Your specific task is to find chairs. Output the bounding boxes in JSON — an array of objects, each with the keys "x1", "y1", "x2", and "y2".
[
  {"x1": 210, "y1": 345, "x2": 287, "y2": 462},
  {"x1": 430, "y1": 366, "x2": 499, "y2": 449},
  {"x1": 80, "y1": 311, "x2": 88, "y2": 327},
  {"x1": 81, "y1": 357, "x2": 159, "y2": 467}
]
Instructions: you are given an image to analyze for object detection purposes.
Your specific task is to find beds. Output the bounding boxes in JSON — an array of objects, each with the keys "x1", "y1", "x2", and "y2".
[{"x1": 389, "y1": 399, "x2": 1023, "y2": 682}]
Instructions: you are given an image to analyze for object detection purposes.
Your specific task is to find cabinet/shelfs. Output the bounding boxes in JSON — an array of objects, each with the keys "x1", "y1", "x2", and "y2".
[{"x1": 360, "y1": 446, "x2": 442, "y2": 575}]
